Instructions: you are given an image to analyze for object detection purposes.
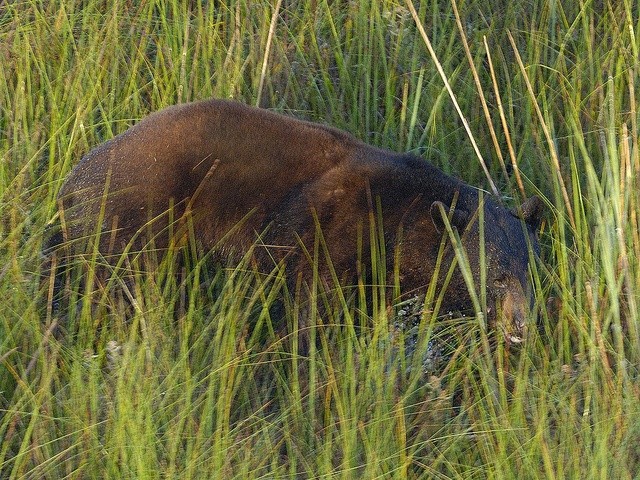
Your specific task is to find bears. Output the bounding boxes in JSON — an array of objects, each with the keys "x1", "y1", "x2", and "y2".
[{"x1": 38, "y1": 99, "x2": 545, "y2": 420}]
